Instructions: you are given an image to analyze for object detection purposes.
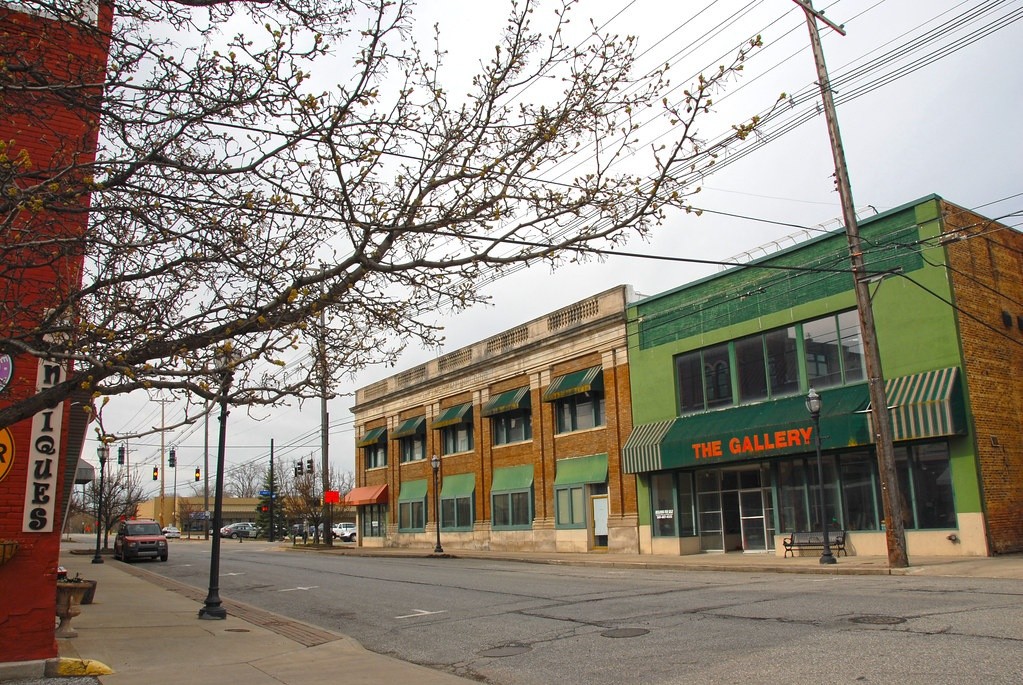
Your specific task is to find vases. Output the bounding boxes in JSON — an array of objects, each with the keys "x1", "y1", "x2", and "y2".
[{"x1": 80, "y1": 580, "x2": 99, "y2": 604}]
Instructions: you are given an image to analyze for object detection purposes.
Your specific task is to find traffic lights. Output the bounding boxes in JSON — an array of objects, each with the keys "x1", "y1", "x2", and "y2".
[
  {"x1": 195, "y1": 469, "x2": 200, "y2": 481},
  {"x1": 307, "y1": 459, "x2": 313, "y2": 474},
  {"x1": 153, "y1": 468, "x2": 158, "y2": 480},
  {"x1": 297, "y1": 462, "x2": 304, "y2": 476}
]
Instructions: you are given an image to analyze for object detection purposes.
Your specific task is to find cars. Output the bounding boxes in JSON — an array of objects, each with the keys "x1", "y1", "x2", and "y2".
[
  {"x1": 294, "y1": 522, "x2": 356, "y2": 541},
  {"x1": 162, "y1": 526, "x2": 181, "y2": 539},
  {"x1": 220, "y1": 522, "x2": 262, "y2": 540},
  {"x1": 114, "y1": 520, "x2": 168, "y2": 563}
]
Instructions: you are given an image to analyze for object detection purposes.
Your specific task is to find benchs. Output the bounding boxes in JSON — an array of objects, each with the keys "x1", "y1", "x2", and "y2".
[{"x1": 781, "y1": 530, "x2": 847, "y2": 558}]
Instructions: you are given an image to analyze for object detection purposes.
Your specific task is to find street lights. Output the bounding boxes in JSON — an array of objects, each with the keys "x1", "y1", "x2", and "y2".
[
  {"x1": 199, "y1": 338, "x2": 241, "y2": 621},
  {"x1": 804, "y1": 387, "x2": 838, "y2": 565},
  {"x1": 431, "y1": 454, "x2": 444, "y2": 553},
  {"x1": 91, "y1": 442, "x2": 109, "y2": 564}
]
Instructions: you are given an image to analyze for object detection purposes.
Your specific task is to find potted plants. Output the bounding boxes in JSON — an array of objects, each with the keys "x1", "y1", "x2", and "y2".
[{"x1": 52, "y1": 571, "x2": 93, "y2": 639}]
